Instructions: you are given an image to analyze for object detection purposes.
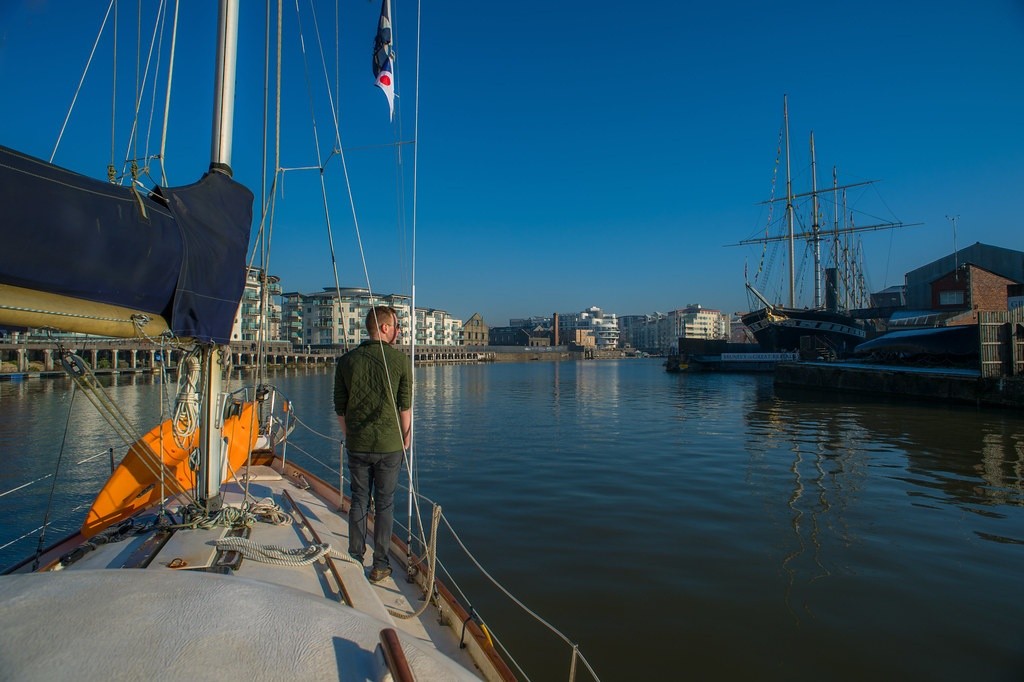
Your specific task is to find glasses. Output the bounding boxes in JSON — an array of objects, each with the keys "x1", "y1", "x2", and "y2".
[{"x1": 379, "y1": 323, "x2": 400, "y2": 329}]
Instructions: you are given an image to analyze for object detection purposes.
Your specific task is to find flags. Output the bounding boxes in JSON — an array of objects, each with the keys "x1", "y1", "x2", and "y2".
[{"x1": 372, "y1": 0, "x2": 395, "y2": 123}]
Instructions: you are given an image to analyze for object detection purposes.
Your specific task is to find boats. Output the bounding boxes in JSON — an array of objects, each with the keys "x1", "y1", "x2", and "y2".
[{"x1": 661, "y1": 327, "x2": 800, "y2": 374}]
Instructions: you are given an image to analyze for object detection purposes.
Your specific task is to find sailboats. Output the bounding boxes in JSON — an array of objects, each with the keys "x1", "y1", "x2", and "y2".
[{"x1": 721, "y1": 93, "x2": 925, "y2": 360}]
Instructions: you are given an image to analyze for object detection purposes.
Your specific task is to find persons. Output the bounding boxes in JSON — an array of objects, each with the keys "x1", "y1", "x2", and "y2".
[{"x1": 332, "y1": 305, "x2": 413, "y2": 580}]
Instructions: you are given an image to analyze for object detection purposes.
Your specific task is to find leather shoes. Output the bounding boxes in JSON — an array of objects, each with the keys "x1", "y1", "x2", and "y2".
[
  {"x1": 353, "y1": 556, "x2": 364, "y2": 565},
  {"x1": 370, "y1": 567, "x2": 391, "y2": 581}
]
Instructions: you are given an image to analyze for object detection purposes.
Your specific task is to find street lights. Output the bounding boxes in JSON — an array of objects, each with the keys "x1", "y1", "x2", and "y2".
[{"x1": 945, "y1": 214, "x2": 962, "y2": 280}]
